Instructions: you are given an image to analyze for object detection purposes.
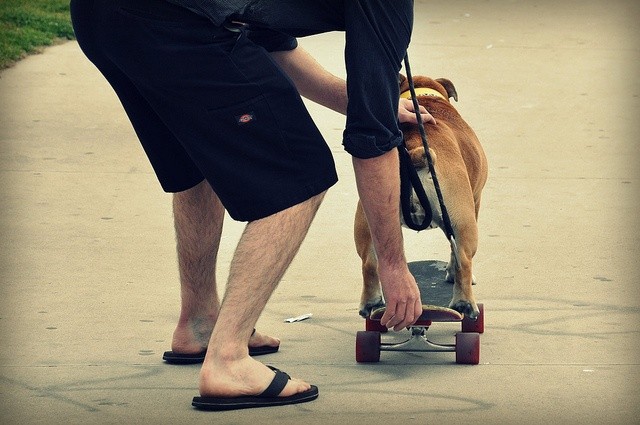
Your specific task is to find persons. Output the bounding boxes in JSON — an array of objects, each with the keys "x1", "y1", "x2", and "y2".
[{"x1": 69, "y1": 0, "x2": 423, "y2": 411}]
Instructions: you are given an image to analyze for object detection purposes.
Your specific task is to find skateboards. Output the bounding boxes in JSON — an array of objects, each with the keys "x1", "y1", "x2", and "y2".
[{"x1": 355, "y1": 260, "x2": 484, "y2": 364}]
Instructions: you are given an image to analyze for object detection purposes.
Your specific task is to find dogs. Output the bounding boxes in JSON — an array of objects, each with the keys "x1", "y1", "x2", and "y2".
[{"x1": 353, "y1": 75, "x2": 490, "y2": 317}]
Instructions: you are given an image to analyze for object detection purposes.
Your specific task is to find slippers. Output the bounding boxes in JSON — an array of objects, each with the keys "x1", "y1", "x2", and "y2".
[
  {"x1": 161, "y1": 326, "x2": 281, "y2": 362},
  {"x1": 191, "y1": 363, "x2": 320, "y2": 410}
]
